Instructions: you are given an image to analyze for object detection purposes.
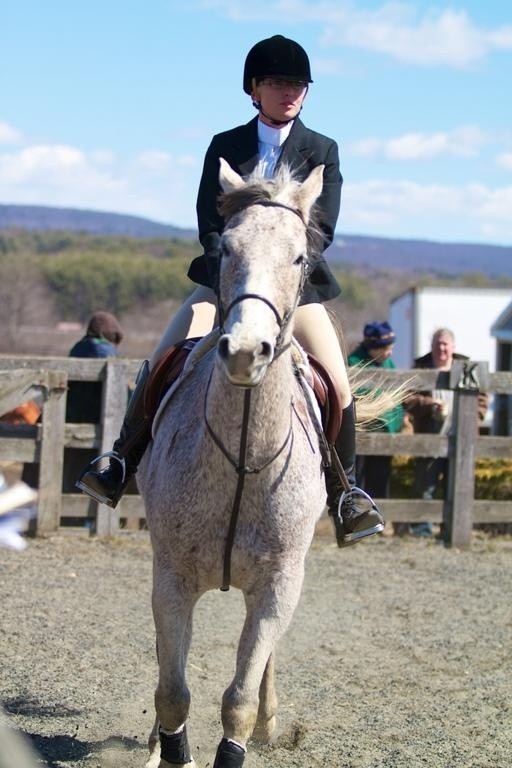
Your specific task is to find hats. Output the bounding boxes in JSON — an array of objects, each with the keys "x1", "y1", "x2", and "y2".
[{"x1": 361, "y1": 318, "x2": 398, "y2": 349}]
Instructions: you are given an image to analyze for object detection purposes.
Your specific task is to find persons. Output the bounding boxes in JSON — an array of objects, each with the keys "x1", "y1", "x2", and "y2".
[
  {"x1": 342, "y1": 314, "x2": 410, "y2": 512},
  {"x1": 27, "y1": 309, "x2": 149, "y2": 496},
  {"x1": 401, "y1": 324, "x2": 490, "y2": 536},
  {"x1": 73, "y1": 34, "x2": 383, "y2": 553}
]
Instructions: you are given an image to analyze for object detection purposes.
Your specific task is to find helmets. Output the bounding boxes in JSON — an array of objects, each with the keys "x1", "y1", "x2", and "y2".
[{"x1": 242, "y1": 33, "x2": 314, "y2": 95}]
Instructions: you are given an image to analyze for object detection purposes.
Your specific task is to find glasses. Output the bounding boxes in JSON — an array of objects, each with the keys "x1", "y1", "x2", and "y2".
[{"x1": 260, "y1": 79, "x2": 309, "y2": 91}]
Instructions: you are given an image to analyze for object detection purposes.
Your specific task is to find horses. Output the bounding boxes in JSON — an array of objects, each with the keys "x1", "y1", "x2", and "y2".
[{"x1": 135, "y1": 157, "x2": 422, "y2": 768}]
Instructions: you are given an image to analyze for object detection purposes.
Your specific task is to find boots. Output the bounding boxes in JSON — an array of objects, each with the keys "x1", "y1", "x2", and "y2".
[
  {"x1": 326, "y1": 400, "x2": 388, "y2": 550},
  {"x1": 71, "y1": 360, "x2": 155, "y2": 510}
]
